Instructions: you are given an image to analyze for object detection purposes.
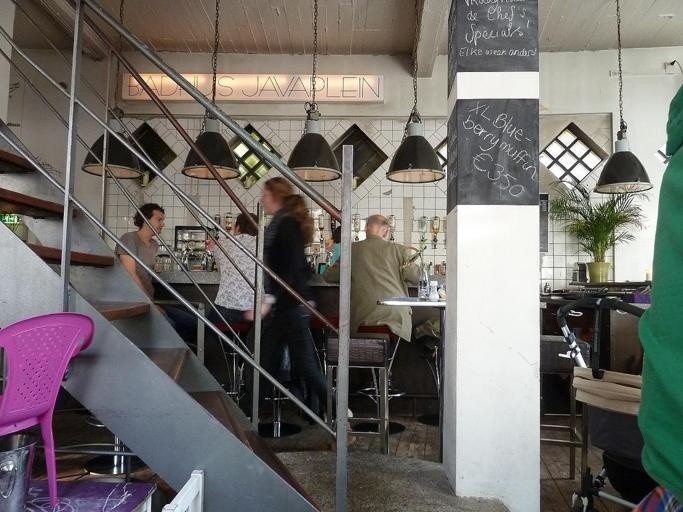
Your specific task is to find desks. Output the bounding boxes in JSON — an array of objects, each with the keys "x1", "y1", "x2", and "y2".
[{"x1": 24, "y1": 478, "x2": 157, "y2": 511}]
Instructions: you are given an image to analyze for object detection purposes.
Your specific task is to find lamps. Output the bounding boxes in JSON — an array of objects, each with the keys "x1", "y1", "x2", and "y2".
[
  {"x1": 386, "y1": 1, "x2": 446, "y2": 184},
  {"x1": 592, "y1": 1, "x2": 654, "y2": 194},
  {"x1": 285, "y1": 1, "x2": 342, "y2": 183},
  {"x1": 179, "y1": 1, "x2": 240, "y2": 181},
  {"x1": 82, "y1": 1, "x2": 143, "y2": 180}
]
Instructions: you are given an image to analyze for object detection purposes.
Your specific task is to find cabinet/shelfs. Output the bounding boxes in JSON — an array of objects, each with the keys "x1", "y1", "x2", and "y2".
[{"x1": 173, "y1": 225, "x2": 217, "y2": 272}]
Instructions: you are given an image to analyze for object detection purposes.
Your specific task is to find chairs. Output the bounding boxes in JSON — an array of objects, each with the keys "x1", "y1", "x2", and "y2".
[
  {"x1": 161, "y1": 467, "x2": 206, "y2": 512},
  {"x1": 0, "y1": 310, "x2": 95, "y2": 508}
]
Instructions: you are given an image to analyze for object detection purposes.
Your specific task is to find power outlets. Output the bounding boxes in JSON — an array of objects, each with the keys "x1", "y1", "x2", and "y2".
[{"x1": 664, "y1": 61, "x2": 676, "y2": 75}]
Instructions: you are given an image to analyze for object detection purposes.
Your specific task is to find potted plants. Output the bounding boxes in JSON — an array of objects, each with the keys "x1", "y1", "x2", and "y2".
[{"x1": 542, "y1": 179, "x2": 647, "y2": 282}]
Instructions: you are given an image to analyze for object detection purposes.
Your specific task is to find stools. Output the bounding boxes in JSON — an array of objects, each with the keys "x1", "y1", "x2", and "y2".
[
  {"x1": 79, "y1": 411, "x2": 146, "y2": 478},
  {"x1": 538, "y1": 334, "x2": 589, "y2": 490},
  {"x1": 207, "y1": 316, "x2": 443, "y2": 459}
]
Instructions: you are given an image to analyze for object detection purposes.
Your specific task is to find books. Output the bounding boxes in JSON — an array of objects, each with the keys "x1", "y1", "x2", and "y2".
[{"x1": 571, "y1": 363, "x2": 642, "y2": 418}]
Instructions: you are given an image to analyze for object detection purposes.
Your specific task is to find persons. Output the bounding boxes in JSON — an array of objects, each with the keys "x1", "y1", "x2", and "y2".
[
  {"x1": 329, "y1": 226, "x2": 341, "y2": 266},
  {"x1": 240, "y1": 176, "x2": 354, "y2": 426},
  {"x1": 209, "y1": 211, "x2": 265, "y2": 392},
  {"x1": 112, "y1": 201, "x2": 200, "y2": 356},
  {"x1": 322, "y1": 213, "x2": 422, "y2": 394}
]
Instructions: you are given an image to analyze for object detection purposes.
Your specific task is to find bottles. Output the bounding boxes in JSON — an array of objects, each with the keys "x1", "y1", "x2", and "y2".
[
  {"x1": 353, "y1": 214, "x2": 362, "y2": 242},
  {"x1": 387, "y1": 214, "x2": 397, "y2": 241},
  {"x1": 318, "y1": 214, "x2": 325, "y2": 241},
  {"x1": 225, "y1": 212, "x2": 233, "y2": 234},
  {"x1": 213, "y1": 215, "x2": 221, "y2": 238},
  {"x1": 430, "y1": 216, "x2": 441, "y2": 248},
  {"x1": 417, "y1": 265, "x2": 429, "y2": 299},
  {"x1": 429, "y1": 281, "x2": 441, "y2": 302},
  {"x1": 418, "y1": 215, "x2": 428, "y2": 247}
]
